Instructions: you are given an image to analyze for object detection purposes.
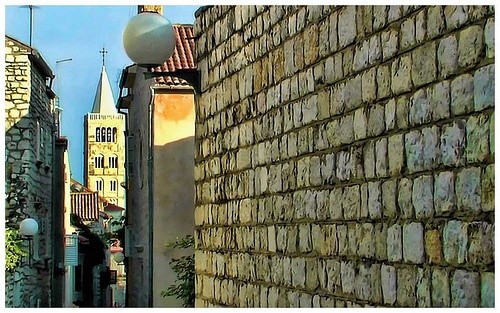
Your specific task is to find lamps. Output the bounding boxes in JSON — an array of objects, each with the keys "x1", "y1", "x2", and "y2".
[
  {"x1": 122, "y1": 9, "x2": 203, "y2": 98},
  {"x1": 14, "y1": 217, "x2": 38, "y2": 241}
]
